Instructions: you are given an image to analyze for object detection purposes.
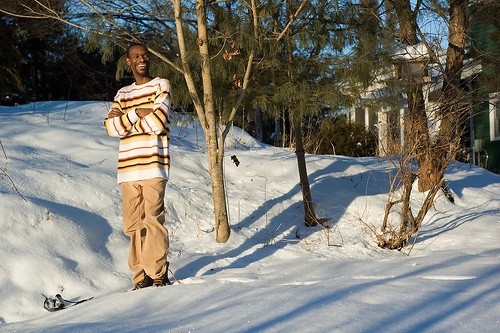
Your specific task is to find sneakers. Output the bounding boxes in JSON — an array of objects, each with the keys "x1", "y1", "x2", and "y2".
[
  {"x1": 153, "y1": 277, "x2": 170, "y2": 286},
  {"x1": 132, "y1": 276, "x2": 152, "y2": 291}
]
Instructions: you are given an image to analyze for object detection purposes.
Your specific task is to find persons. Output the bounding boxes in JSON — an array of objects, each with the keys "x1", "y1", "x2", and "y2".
[{"x1": 103, "y1": 44, "x2": 174, "y2": 290}]
